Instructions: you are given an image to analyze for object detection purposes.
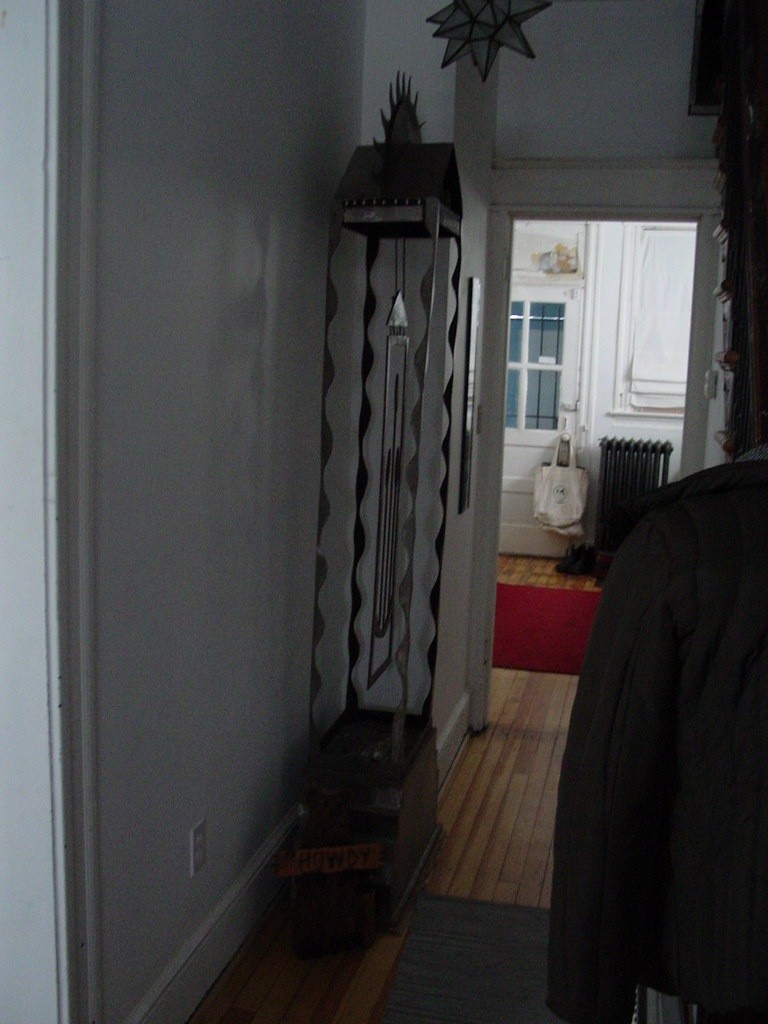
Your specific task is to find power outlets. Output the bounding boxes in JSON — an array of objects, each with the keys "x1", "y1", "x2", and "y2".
[{"x1": 189, "y1": 818, "x2": 207, "y2": 875}]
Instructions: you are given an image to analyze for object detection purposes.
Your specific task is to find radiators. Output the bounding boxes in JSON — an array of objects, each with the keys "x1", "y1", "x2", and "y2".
[{"x1": 595, "y1": 436, "x2": 672, "y2": 575}]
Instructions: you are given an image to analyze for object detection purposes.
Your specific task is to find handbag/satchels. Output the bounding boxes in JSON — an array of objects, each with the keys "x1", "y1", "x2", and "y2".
[{"x1": 533, "y1": 431, "x2": 587, "y2": 527}]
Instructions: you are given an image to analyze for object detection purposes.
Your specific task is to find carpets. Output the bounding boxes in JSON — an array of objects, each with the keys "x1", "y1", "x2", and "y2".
[
  {"x1": 493, "y1": 584, "x2": 602, "y2": 675},
  {"x1": 380, "y1": 893, "x2": 558, "y2": 1024}
]
